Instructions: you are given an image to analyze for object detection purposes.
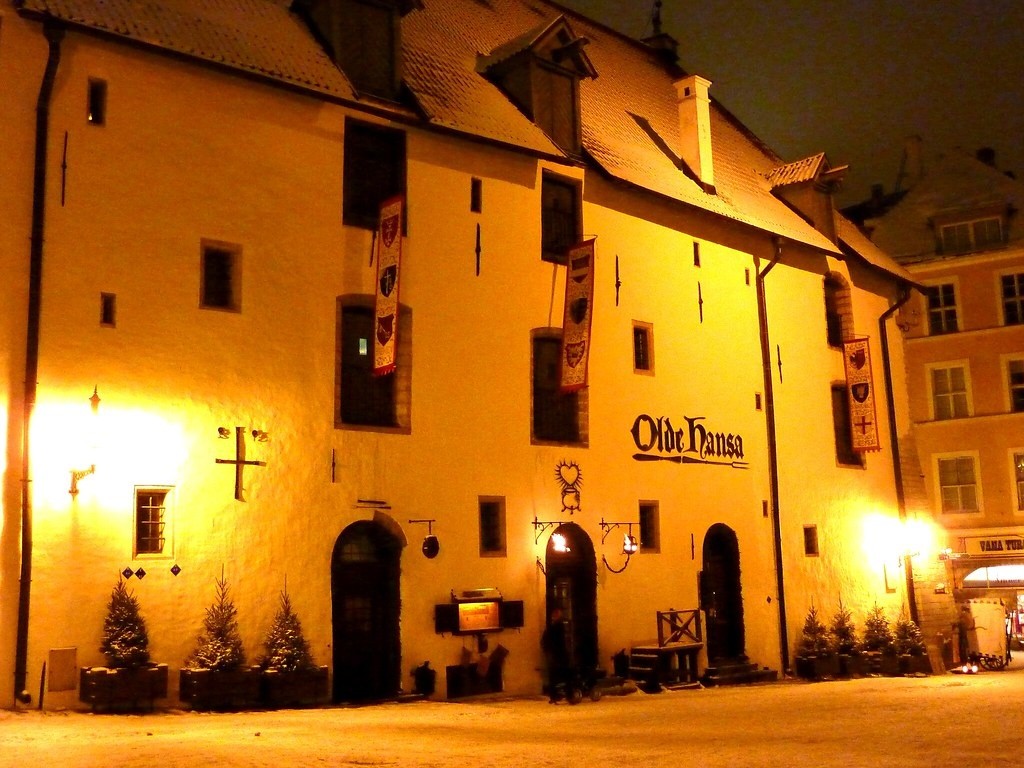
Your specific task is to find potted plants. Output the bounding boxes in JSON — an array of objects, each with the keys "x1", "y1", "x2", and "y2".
[
  {"x1": 795, "y1": 592, "x2": 927, "y2": 682},
  {"x1": 80, "y1": 568, "x2": 168, "y2": 702},
  {"x1": 180, "y1": 563, "x2": 321, "y2": 710}
]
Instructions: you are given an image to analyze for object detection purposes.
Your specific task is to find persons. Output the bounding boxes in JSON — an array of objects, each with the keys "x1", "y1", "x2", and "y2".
[{"x1": 541, "y1": 606, "x2": 572, "y2": 701}]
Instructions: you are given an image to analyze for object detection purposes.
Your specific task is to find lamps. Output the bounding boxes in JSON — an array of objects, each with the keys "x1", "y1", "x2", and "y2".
[
  {"x1": 599, "y1": 517, "x2": 642, "y2": 574},
  {"x1": 531, "y1": 517, "x2": 574, "y2": 580},
  {"x1": 408, "y1": 520, "x2": 439, "y2": 559}
]
